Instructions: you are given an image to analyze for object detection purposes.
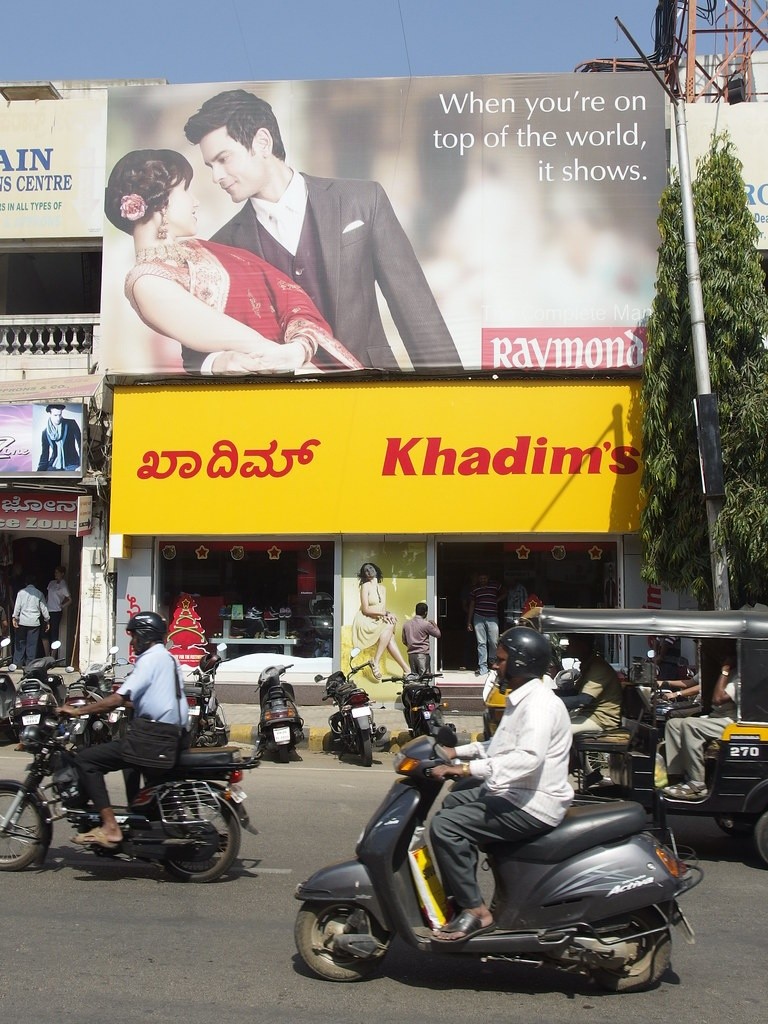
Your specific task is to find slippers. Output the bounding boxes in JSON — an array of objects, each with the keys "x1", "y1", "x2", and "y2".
[
  {"x1": 69, "y1": 826, "x2": 120, "y2": 849},
  {"x1": 430, "y1": 912, "x2": 496, "y2": 945}
]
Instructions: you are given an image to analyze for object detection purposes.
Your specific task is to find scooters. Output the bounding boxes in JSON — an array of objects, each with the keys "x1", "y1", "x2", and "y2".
[{"x1": 284, "y1": 709, "x2": 704, "y2": 996}]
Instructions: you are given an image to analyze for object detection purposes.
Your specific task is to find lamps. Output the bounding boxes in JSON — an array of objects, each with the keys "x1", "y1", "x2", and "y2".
[{"x1": 727, "y1": 69, "x2": 746, "y2": 106}]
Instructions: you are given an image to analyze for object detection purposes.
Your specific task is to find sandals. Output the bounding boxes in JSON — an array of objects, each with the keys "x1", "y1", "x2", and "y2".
[
  {"x1": 661, "y1": 784, "x2": 680, "y2": 792},
  {"x1": 667, "y1": 781, "x2": 708, "y2": 800}
]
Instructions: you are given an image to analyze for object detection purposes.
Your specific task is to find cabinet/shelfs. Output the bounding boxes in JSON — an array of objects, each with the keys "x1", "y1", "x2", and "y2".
[{"x1": 209, "y1": 612, "x2": 300, "y2": 658}]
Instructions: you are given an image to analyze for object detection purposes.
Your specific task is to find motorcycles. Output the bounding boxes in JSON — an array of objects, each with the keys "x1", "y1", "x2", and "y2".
[
  {"x1": 312, "y1": 647, "x2": 387, "y2": 767},
  {"x1": 9, "y1": 639, "x2": 66, "y2": 750},
  {"x1": 254, "y1": 662, "x2": 304, "y2": 763},
  {"x1": 0, "y1": 682, "x2": 264, "y2": 882},
  {"x1": 178, "y1": 653, "x2": 230, "y2": 750},
  {"x1": 527, "y1": 606, "x2": 768, "y2": 867},
  {"x1": 0, "y1": 637, "x2": 18, "y2": 730},
  {"x1": 108, "y1": 638, "x2": 175, "y2": 742},
  {"x1": 381, "y1": 670, "x2": 457, "y2": 741},
  {"x1": 63, "y1": 645, "x2": 121, "y2": 750}
]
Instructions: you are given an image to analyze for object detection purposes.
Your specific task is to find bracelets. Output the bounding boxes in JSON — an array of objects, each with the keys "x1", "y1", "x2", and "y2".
[
  {"x1": 461, "y1": 762, "x2": 470, "y2": 776},
  {"x1": 75, "y1": 708, "x2": 81, "y2": 715},
  {"x1": 676, "y1": 690, "x2": 682, "y2": 698}
]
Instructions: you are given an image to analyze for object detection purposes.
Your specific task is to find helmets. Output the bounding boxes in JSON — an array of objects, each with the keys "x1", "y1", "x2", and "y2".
[
  {"x1": 199, "y1": 654, "x2": 221, "y2": 674},
  {"x1": 498, "y1": 626, "x2": 552, "y2": 681},
  {"x1": 126, "y1": 612, "x2": 167, "y2": 655}
]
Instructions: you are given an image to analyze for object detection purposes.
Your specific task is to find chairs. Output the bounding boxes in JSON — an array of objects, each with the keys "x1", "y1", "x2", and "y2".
[{"x1": 572, "y1": 686, "x2": 651, "y2": 794}]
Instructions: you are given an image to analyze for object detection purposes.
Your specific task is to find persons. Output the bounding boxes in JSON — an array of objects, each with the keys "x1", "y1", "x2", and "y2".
[
  {"x1": 36, "y1": 405, "x2": 86, "y2": 472},
  {"x1": 54, "y1": 611, "x2": 193, "y2": 849},
  {"x1": 426, "y1": 625, "x2": 572, "y2": 942},
  {"x1": 660, "y1": 650, "x2": 740, "y2": 801},
  {"x1": 7, "y1": 561, "x2": 23, "y2": 612},
  {"x1": 652, "y1": 670, "x2": 702, "y2": 724},
  {"x1": 105, "y1": 88, "x2": 467, "y2": 375},
  {"x1": 353, "y1": 563, "x2": 414, "y2": 679},
  {"x1": 12, "y1": 575, "x2": 51, "y2": 668},
  {"x1": 466, "y1": 569, "x2": 529, "y2": 674},
  {"x1": 402, "y1": 603, "x2": 441, "y2": 685},
  {"x1": 650, "y1": 636, "x2": 683, "y2": 680},
  {"x1": 565, "y1": 638, "x2": 624, "y2": 738},
  {"x1": 41, "y1": 564, "x2": 72, "y2": 660},
  {"x1": 0, "y1": 605, "x2": 7, "y2": 657}
]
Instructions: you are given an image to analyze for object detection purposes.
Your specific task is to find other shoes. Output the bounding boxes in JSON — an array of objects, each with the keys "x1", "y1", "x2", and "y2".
[{"x1": 583, "y1": 768, "x2": 603, "y2": 788}]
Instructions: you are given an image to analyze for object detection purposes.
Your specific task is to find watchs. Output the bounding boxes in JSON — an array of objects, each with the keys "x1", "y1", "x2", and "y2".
[{"x1": 722, "y1": 671, "x2": 729, "y2": 675}]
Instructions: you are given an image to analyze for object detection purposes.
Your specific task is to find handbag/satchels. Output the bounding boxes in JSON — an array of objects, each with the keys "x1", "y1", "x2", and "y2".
[
  {"x1": 408, "y1": 817, "x2": 456, "y2": 930},
  {"x1": 122, "y1": 718, "x2": 187, "y2": 771}
]
